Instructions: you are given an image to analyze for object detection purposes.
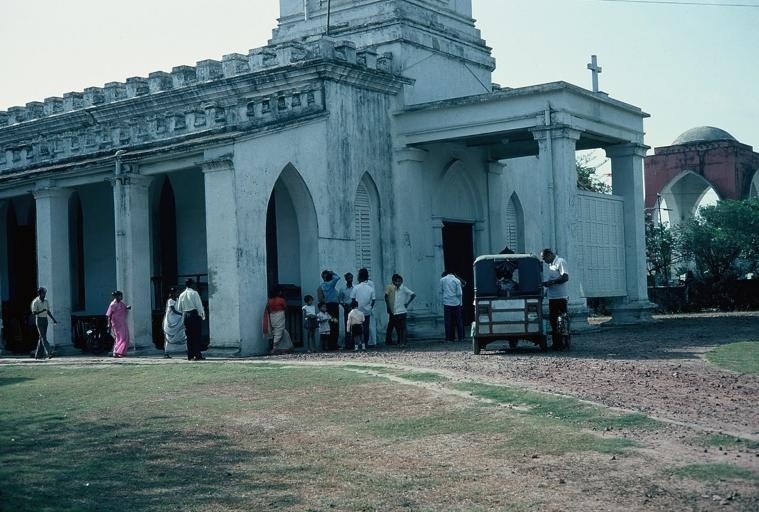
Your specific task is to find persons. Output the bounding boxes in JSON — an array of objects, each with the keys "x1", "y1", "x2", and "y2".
[
  {"x1": 105, "y1": 291, "x2": 131, "y2": 358},
  {"x1": 302, "y1": 268, "x2": 416, "y2": 351},
  {"x1": 163, "y1": 287, "x2": 188, "y2": 358},
  {"x1": 177, "y1": 278, "x2": 205, "y2": 361},
  {"x1": 30, "y1": 287, "x2": 57, "y2": 359},
  {"x1": 541, "y1": 248, "x2": 569, "y2": 349},
  {"x1": 263, "y1": 287, "x2": 289, "y2": 353},
  {"x1": 440, "y1": 270, "x2": 467, "y2": 342}
]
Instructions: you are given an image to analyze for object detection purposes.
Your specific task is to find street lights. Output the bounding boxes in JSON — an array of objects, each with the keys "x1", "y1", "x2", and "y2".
[{"x1": 644, "y1": 192, "x2": 674, "y2": 285}]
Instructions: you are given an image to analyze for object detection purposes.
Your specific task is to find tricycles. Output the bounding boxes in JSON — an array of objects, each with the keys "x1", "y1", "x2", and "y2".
[{"x1": 470, "y1": 253, "x2": 548, "y2": 355}]
